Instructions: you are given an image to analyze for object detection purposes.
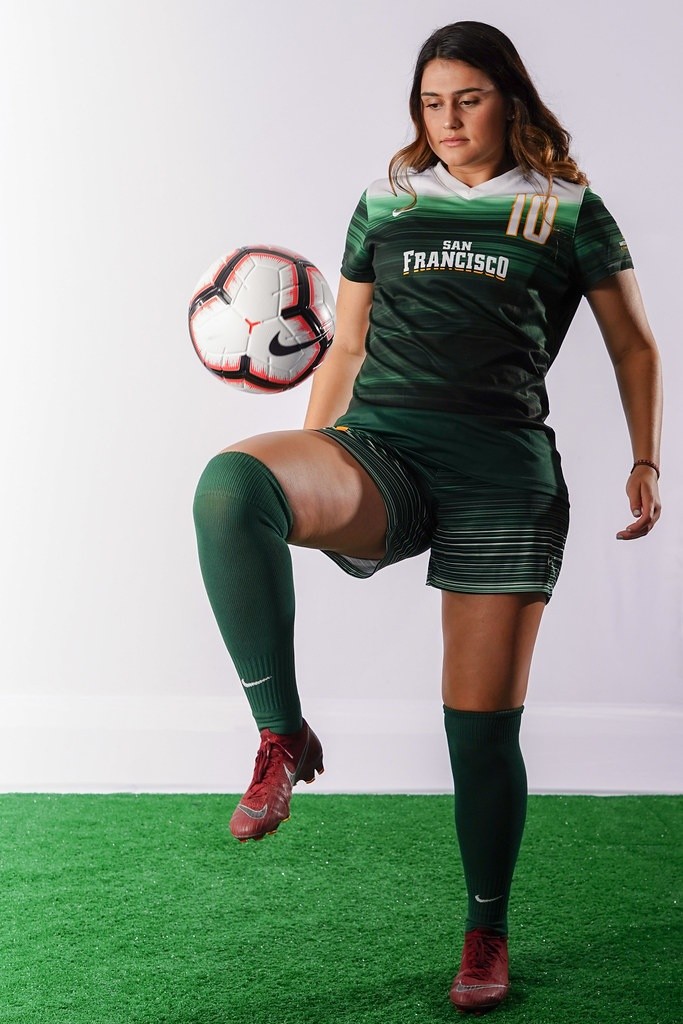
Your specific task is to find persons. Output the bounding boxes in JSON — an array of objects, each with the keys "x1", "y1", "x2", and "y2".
[{"x1": 193, "y1": 21, "x2": 663, "y2": 1013}]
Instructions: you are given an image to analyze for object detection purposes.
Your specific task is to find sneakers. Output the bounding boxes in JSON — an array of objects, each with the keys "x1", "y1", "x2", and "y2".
[
  {"x1": 231, "y1": 718, "x2": 325, "y2": 842},
  {"x1": 451, "y1": 927, "x2": 509, "y2": 1011}
]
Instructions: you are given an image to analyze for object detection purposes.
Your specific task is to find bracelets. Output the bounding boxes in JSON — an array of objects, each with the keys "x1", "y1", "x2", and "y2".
[{"x1": 630, "y1": 459, "x2": 660, "y2": 479}]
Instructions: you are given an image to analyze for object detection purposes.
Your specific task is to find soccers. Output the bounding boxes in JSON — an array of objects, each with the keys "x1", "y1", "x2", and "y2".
[{"x1": 186, "y1": 242, "x2": 338, "y2": 396}]
